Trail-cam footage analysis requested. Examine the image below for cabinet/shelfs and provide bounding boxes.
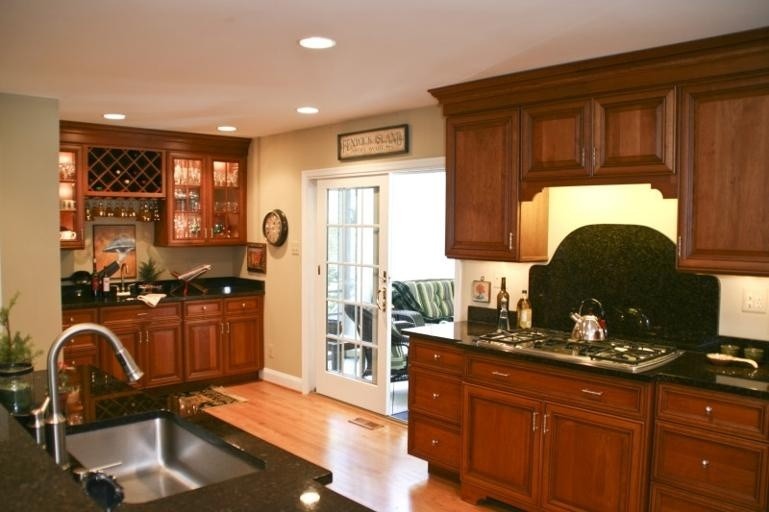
[57,120,253,248]
[99,302,184,394]
[591,83,677,184]
[444,108,548,263]
[407,333,463,483]
[519,97,592,186]
[183,292,264,391]
[675,73,769,278]
[62,307,99,372]
[649,380,769,512]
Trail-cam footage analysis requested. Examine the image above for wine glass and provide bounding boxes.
[85,198,162,224]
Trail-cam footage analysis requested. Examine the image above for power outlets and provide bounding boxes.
[742,295,763,312]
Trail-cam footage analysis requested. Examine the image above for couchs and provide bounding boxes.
[392,278,455,333]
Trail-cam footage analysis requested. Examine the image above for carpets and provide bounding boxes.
[177,382,247,409]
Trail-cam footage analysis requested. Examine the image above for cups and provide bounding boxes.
[62,199,77,209]
[743,347,764,365]
[60,230,76,240]
[175,160,232,187]
[722,343,740,356]
[177,190,238,212]
[173,214,232,238]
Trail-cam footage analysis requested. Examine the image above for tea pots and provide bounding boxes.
[568,297,609,343]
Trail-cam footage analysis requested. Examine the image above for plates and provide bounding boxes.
[103,245,136,253]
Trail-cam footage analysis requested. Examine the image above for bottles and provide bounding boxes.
[498,297,511,330]
[102,265,112,297]
[517,289,533,329]
[497,277,510,316]
[91,259,100,297]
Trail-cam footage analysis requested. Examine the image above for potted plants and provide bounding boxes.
[0,288,44,413]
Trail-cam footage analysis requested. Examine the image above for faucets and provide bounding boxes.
[44,321,144,471]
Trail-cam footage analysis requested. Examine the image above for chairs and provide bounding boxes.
[327,320,360,370]
[461,351,654,511]
[345,305,410,383]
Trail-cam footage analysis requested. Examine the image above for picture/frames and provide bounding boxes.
[472,280,491,304]
[337,123,409,162]
[93,224,137,280]
[246,243,266,274]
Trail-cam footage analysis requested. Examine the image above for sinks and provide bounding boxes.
[64,409,266,504]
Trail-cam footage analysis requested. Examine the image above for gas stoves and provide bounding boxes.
[472,325,686,376]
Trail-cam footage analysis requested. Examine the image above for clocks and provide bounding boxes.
[262,209,288,246]
